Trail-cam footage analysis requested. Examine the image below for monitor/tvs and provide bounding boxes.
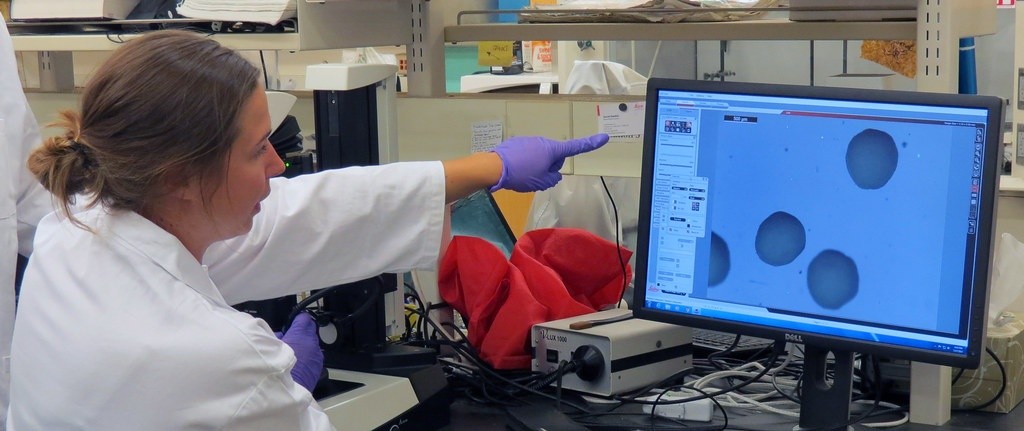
[632,76,1007,431]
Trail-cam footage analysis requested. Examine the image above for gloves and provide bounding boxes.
[487,134,609,193]
[273,313,324,394]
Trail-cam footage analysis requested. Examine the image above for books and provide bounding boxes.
[176,0,297,25]
[11,1,142,20]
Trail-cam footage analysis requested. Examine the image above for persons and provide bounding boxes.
[0,9,609,431]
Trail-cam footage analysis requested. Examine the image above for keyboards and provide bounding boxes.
[690,327,777,361]
